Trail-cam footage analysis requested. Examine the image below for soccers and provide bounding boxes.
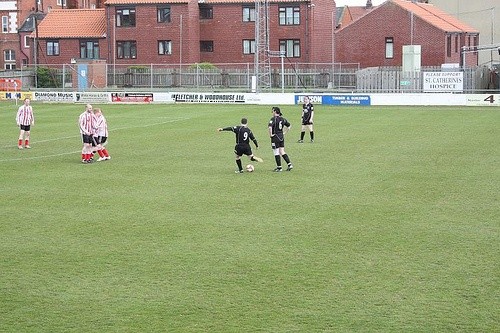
[246,163,255,172]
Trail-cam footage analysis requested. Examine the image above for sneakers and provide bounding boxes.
[252,156,263,163]
[235,169,244,173]
[285,164,293,171]
[273,167,282,172]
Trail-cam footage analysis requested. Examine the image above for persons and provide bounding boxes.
[268,107,293,172]
[16,98,35,150]
[77,104,111,164]
[296,97,315,143]
[217,117,263,174]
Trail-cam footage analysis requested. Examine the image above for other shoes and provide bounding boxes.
[311,139,314,143]
[97,156,105,162]
[297,140,303,143]
[87,159,91,163]
[90,158,93,161]
[82,159,86,162]
[18,146,23,149]
[25,146,31,148]
[105,156,110,160]
